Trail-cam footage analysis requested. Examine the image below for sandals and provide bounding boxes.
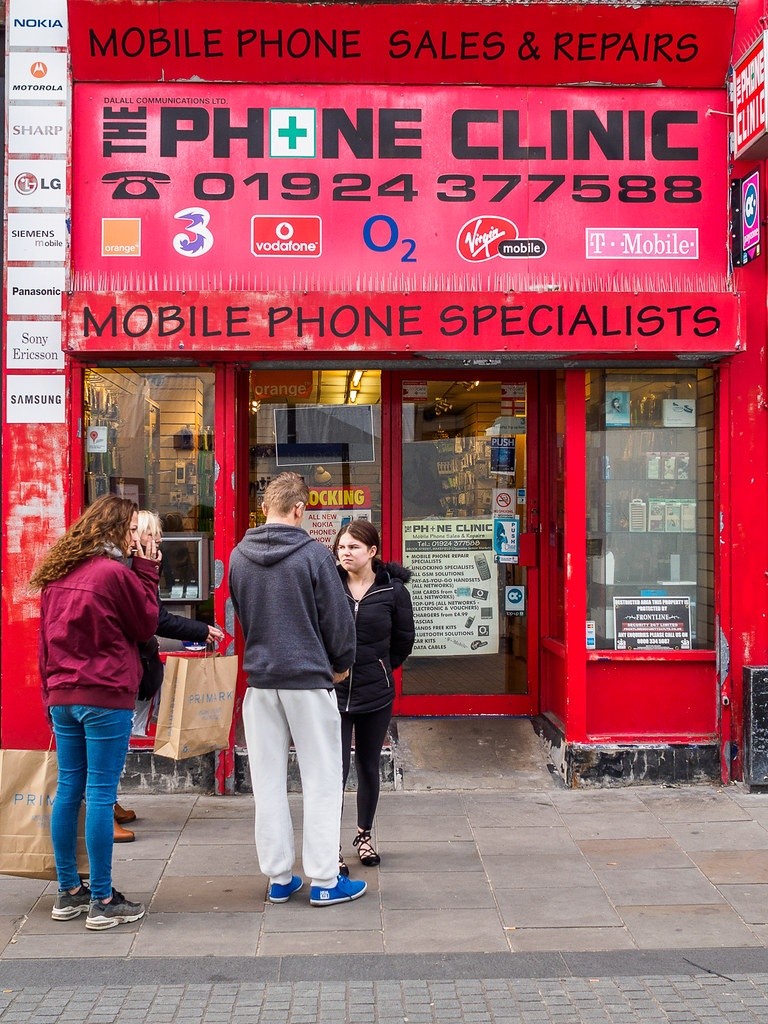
[339,853,349,877]
[353,829,380,866]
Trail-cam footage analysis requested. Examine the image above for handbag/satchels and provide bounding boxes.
[153,640,241,760]
[0,730,89,881]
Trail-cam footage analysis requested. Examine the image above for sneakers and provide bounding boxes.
[269,876,303,903]
[310,873,367,906]
[86,887,145,930]
[52,882,92,921]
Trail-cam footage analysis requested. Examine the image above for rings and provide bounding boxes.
[151,556,156,558]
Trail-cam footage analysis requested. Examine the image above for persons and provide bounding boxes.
[112,509,225,843]
[28,493,162,930]
[228,471,368,906]
[333,518,416,877]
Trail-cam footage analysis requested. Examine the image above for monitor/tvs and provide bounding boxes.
[156,536,209,601]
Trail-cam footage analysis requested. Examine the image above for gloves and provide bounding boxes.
[137,636,163,701]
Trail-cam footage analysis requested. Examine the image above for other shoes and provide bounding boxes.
[113,818,135,843]
[114,802,136,824]
